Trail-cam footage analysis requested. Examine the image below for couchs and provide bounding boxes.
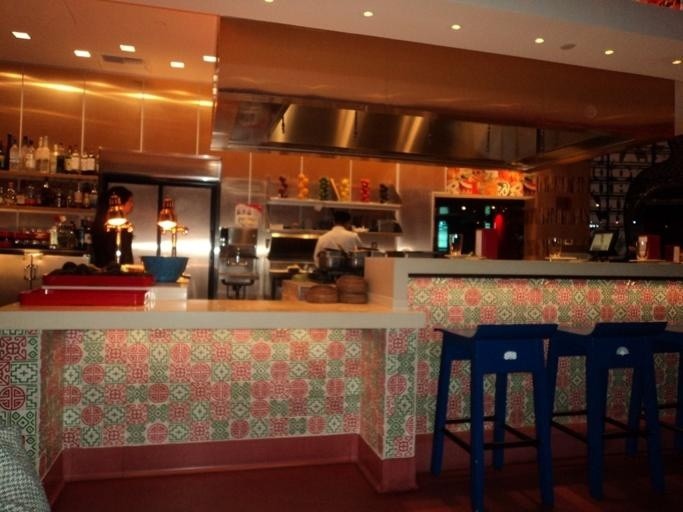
[150,276,187,300]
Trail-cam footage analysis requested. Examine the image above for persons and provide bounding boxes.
[313,210,362,268]
[92,186,136,266]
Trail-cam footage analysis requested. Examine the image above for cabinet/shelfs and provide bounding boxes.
[263,175,404,300]
[0,61,218,169]
[534,175,590,260]
[0,170,99,305]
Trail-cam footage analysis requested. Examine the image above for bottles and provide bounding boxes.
[0,130,100,176]
[0,213,96,252]
[0,179,99,209]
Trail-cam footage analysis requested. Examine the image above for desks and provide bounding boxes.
[282,280,335,300]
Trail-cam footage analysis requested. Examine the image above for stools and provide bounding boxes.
[626,330,683,457]
[545,322,668,501]
[430,324,559,512]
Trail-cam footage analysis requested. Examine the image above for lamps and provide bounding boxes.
[103,192,134,263]
[156,197,191,280]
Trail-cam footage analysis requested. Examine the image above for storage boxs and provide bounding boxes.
[18,288,148,306]
[41,273,155,286]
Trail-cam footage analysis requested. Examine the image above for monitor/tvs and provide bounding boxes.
[588,229,619,257]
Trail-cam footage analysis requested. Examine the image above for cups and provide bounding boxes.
[448,233,464,256]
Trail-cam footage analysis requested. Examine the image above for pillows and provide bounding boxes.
[0,423,51,512]
